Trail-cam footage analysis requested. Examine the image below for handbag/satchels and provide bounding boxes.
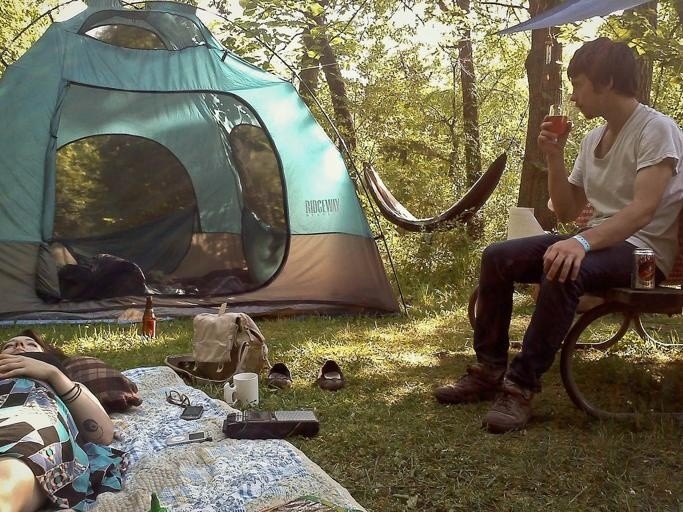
[193,303,268,380]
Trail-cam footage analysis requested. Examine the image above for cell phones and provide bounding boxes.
[180,406,203,420]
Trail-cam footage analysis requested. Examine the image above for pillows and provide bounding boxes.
[62,357,140,414]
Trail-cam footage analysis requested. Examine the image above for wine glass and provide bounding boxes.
[548,104,570,145]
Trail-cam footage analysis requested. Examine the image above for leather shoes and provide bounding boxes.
[318,359,343,390]
[267,363,293,389]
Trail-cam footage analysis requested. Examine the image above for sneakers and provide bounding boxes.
[483,380,535,431]
[435,362,506,404]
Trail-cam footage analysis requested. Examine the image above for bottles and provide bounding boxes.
[142,295,157,339]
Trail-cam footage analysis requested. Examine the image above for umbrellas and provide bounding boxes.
[489,0,655,35]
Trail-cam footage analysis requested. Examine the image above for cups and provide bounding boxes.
[223,372,259,409]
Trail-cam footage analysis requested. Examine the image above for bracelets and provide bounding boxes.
[571,235,591,254]
[60,383,82,404]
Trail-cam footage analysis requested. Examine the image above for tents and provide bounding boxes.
[0,0,401,323]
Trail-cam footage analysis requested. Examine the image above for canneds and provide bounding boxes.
[632,248,656,290]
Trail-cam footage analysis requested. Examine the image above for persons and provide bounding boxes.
[0,328,114,512]
[433,38,683,434]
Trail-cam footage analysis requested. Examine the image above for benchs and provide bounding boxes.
[468,279,680,423]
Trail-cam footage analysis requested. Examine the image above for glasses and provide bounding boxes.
[165,391,189,408]
